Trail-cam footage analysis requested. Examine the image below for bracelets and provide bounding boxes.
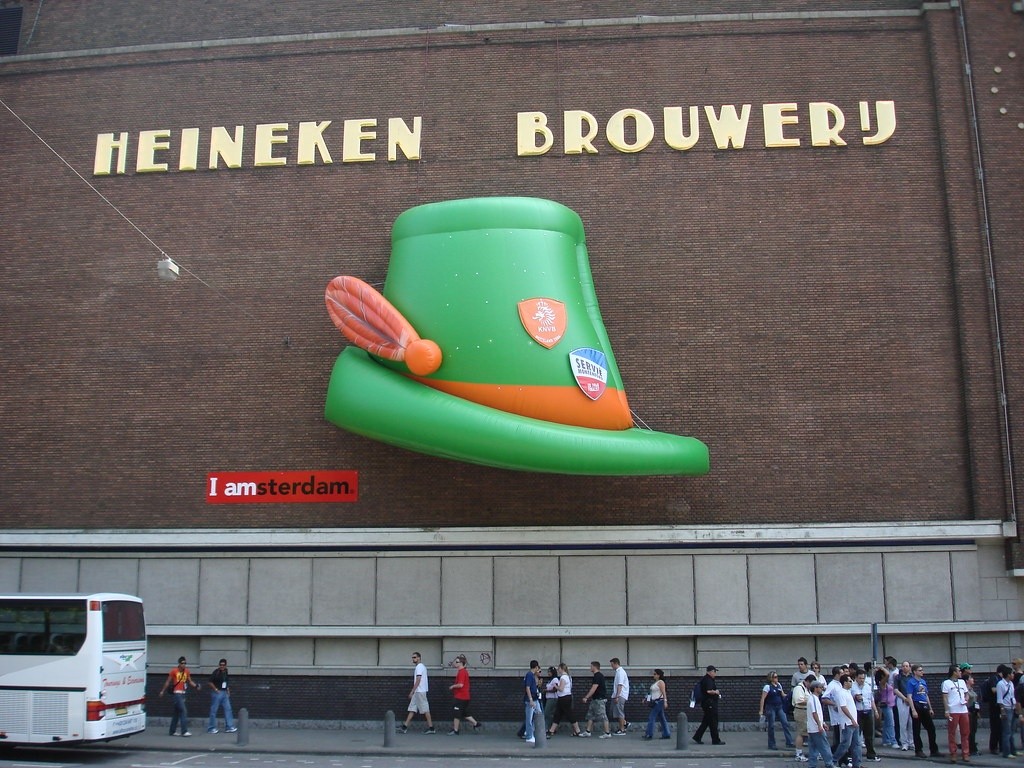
[215,688,218,692]
[585,696,589,699]
[664,699,667,701]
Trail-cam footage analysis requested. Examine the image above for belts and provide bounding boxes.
[795,707,807,710]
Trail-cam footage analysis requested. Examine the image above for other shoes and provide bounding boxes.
[525,722,726,745]
[182,732,192,737]
[517,731,526,739]
[172,732,179,735]
[763,736,1024,768]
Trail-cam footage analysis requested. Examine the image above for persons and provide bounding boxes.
[986,657,1024,758]
[446,657,482,736]
[523,660,543,742]
[609,658,632,735]
[642,669,670,739]
[546,662,580,736]
[894,660,946,757]
[160,656,201,737]
[692,665,726,745]
[578,661,612,738]
[517,666,551,739]
[941,662,982,762]
[790,655,900,768]
[395,652,436,735]
[758,670,796,749]
[207,659,238,734]
[544,667,559,734]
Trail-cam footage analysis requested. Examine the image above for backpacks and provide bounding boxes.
[782,685,804,714]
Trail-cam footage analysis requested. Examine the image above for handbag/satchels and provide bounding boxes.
[520,678,526,692]
[168,693,177,705]
[759,714,766,732]
[648,703,655,708]
[689,679,702,708]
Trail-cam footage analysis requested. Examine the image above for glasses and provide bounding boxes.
[552,668,555,670]
[1011,662,1017,666]
[412,657,416,659]
[219,663,226,666]
[918,670,922,671]
[456,661,460,663]
[849,680,852,682]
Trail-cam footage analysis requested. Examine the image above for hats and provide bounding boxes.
[706,665,719,672]
[960,662,973,670]
[179,657,185,662]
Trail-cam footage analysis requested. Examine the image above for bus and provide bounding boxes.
[0,592,147,754]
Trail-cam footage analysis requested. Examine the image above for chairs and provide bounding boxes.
[10,632,74,654]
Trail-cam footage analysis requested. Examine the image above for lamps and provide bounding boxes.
[156,253,180,282]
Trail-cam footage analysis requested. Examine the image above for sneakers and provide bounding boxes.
[424,729,435,734]
[208,729,219,734]
[474,722,482,735]
[446,729,460,736]
[225,726,237,733]
[395,726,408,734]
